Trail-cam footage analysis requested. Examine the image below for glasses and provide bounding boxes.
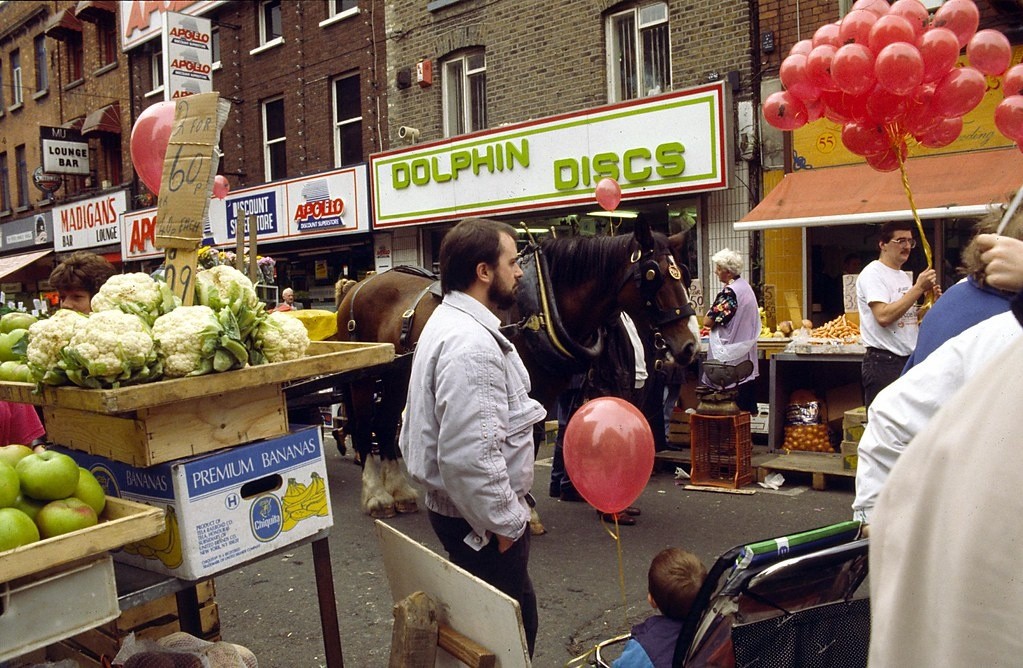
[889,238,917,249]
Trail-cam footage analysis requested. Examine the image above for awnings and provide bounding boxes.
[45,0,115,41]
[59,105,122,136]
[0,248,52,279]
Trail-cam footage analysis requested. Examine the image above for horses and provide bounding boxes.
[335,207,700,534]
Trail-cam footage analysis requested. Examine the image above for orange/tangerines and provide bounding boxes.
[781,398,834,453]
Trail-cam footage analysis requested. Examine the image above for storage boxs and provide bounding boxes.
[45,577,224,668]
[840,440,859,471]
[690,410,751,490]
[53,422,336,582]
[842,406,867,442]
[43,384,290,468]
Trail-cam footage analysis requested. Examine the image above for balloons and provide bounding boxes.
[763,0,1023,173]
[563,397,657,520]
[213,176,229,199]
[595,178,622,211]
[130,101,179,197]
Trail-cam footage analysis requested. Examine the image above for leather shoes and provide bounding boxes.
[598,504,640,525]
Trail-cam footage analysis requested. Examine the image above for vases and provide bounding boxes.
[137,197,151,207]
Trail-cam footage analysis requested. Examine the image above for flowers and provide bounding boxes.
[134,187,153,205]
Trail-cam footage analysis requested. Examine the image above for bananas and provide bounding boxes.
[757,326,772,339]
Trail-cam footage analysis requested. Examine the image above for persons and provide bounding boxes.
[49,251,118,316]
[277,288,303,311]
[0,400,46,450]
[701,248,762,415]
[851,197,1023,668]
[627,53,653,99]
[608,550,707,668]
[663,263,701,451]
[399,215,547,664]
[550,377,641,525]
[331,279,362,465]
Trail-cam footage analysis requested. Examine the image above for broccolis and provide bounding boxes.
[11,264,309,387]
